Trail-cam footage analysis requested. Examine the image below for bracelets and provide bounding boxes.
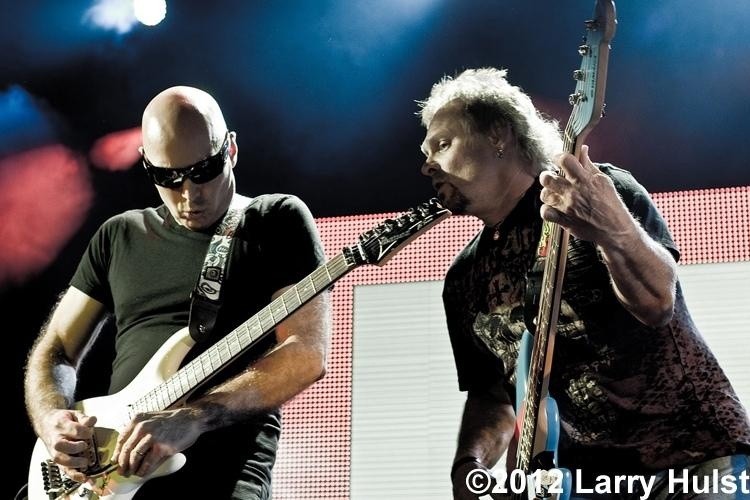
[450,455,482,482]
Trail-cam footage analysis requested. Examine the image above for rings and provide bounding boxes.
[133,448,144,459]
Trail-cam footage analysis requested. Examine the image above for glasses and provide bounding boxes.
[141,128,231,190]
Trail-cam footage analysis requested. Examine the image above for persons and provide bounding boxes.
[417,66,749,500]
[20,85,327,500]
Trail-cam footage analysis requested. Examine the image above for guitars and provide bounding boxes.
[505,2,618,498]
[27,197,452,499]
